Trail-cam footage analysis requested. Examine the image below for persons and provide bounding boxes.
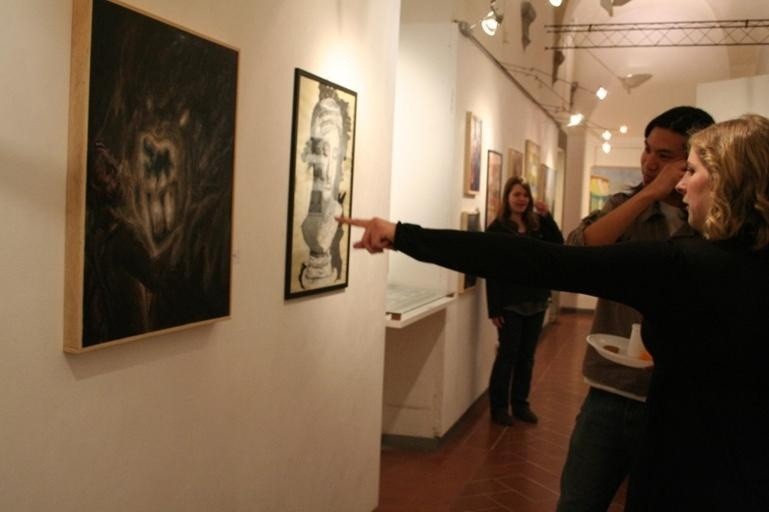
[333,112,769,512]
[482,175,566,426]
[557,104,719,511]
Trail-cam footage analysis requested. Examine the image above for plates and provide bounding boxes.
[585,332,655,368]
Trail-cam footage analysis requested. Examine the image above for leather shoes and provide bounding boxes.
[513,405,537,423]
[493,413,512,426]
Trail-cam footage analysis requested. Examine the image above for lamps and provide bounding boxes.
[585,48,652,93]
[499,61,628,153]
[469,10,502,37]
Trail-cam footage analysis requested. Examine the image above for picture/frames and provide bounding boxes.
[286,68,358,299]
[63,0,240,356]
[458,110,553,294]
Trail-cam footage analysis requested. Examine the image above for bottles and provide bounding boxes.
[628,324,646,358]
